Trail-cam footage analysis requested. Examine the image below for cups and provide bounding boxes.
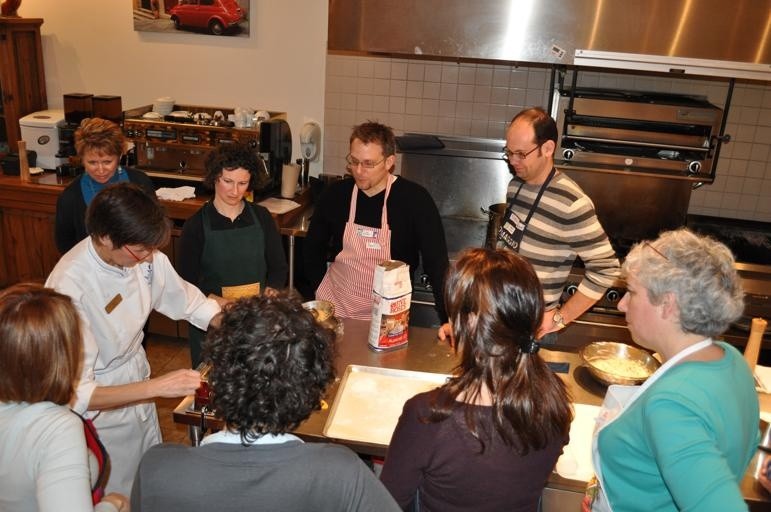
[280,164,300,199]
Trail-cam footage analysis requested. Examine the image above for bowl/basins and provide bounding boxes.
[303,300,336,324]
[579,340,661,388]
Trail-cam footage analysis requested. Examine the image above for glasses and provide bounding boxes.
[642,242,668,260]
[123,245,156,262]
[346,153,390,168]
[502,144,542,159]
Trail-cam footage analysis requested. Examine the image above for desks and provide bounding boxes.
[173,314,771,512]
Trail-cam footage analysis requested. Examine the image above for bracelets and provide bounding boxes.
[99,494,124,510]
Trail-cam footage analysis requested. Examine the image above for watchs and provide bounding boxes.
[554,309,568,329]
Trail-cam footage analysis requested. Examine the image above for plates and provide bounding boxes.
[143,95,176,121]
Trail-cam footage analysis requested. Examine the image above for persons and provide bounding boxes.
[129,296,405,512]
[55,119,159,254]
[380,249,575,512]
[590,227,762,512]
[1,283,126,510]
[296,123,459,350]
[178,146,288,370]
[496,107,623,352]
[44,184,225,510]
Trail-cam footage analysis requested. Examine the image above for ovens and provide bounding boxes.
[560,95,723,187]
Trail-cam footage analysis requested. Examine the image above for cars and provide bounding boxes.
[168,0,246,35]
